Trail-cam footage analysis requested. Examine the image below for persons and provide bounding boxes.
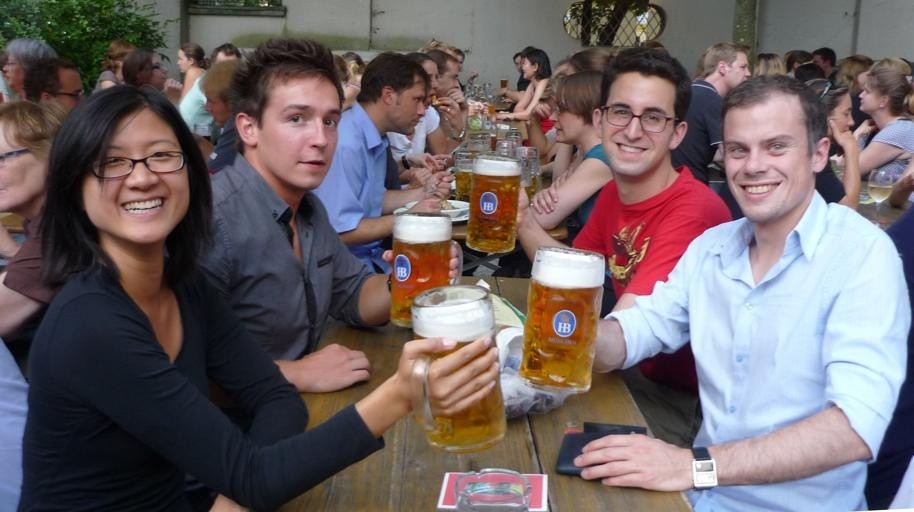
[494,41,913,510]
[0,36,500,512]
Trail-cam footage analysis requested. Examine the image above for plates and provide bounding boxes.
[406,199,470,217]
[394,205,469,222]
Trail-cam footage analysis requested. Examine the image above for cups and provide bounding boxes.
[447,129,543,200]
[465,153,522,254]
[499,78,508,88]
[410,285,507,455]
[389,214,453,328]
[518,245,606,394]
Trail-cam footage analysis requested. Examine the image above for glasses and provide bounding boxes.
[151,62,164,70]
[90,151,186,180]
[51,88,84,101]
[0,148,30,162]
[819,81,834,101]
[554,101,576,115]
[601,104,678,133]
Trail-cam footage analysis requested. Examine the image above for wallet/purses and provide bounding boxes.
[556,422,646,476]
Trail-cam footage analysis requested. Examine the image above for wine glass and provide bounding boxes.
[866,169,894,218]
